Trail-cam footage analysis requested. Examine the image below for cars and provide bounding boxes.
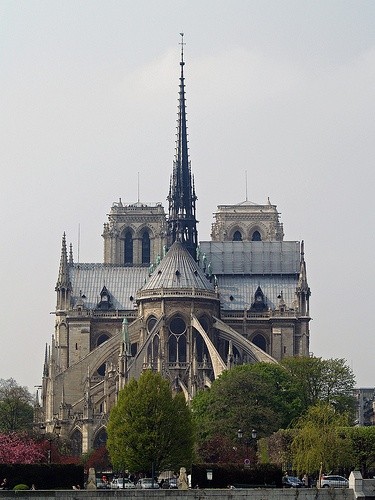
[83,478,110,489]
[109,478,136,489]
[136,478,160,489]
[282,476,305,488]
[162,478,178,489]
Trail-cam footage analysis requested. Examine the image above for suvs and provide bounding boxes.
[320,474,349,488]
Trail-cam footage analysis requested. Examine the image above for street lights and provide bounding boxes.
[38,423,61,463]
[237,429,257,458]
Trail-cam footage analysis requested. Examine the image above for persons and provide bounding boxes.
[160,479,164,488]
[302,474,308,488]
